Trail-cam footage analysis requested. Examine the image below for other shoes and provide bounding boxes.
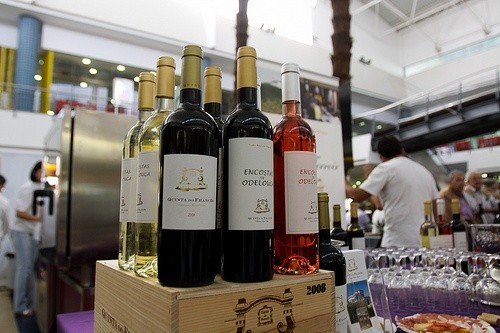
[17,309,32,318]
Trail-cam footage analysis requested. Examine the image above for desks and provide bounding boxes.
[55,301,500,333]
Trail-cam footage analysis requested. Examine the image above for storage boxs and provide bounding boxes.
[94,259,336,333]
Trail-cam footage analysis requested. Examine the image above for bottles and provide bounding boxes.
[220,45,274,284]
[202,67,225,276]
[271,62,320,276]
[419,199,439,266]
[449,198,473,276]
[355,291,373,330]
[118,72,156,272]
[435,199,456,270]
[329,205,350,251]
[156,45,220,288]
[132,55,176,281]
[346,201,366,250]
[318,191,348,333]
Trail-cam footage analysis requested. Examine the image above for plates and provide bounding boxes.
[396,312,496,333]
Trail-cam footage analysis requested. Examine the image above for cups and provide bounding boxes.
[470,224,500,254]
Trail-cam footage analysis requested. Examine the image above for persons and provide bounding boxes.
[346,134,439,266]
[439,170,483,225]
[463,170,498,224]
[0,175,12,241]
[346,202,370,229]
[9,161,43,316]
[369,195,384,233]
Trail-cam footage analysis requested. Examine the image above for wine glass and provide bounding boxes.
[361,246,500,310]
